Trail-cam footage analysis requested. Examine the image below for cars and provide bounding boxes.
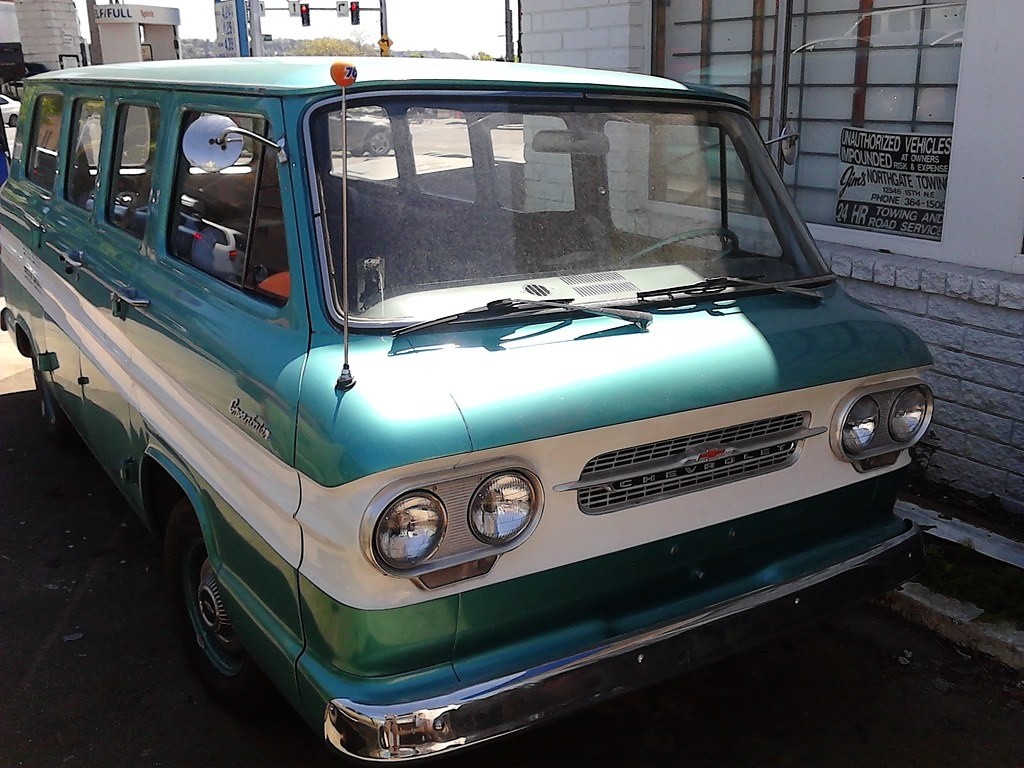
[326,111,396,157]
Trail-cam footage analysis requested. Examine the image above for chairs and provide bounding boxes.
[507,211,625,277]
[209,198,381,293]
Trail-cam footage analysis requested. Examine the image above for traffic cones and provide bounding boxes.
[456,110,460,120]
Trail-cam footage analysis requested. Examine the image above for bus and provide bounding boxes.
[2,51,943,767]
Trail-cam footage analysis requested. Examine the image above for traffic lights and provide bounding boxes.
[351,2,361,25]
[300,4,311,27]
